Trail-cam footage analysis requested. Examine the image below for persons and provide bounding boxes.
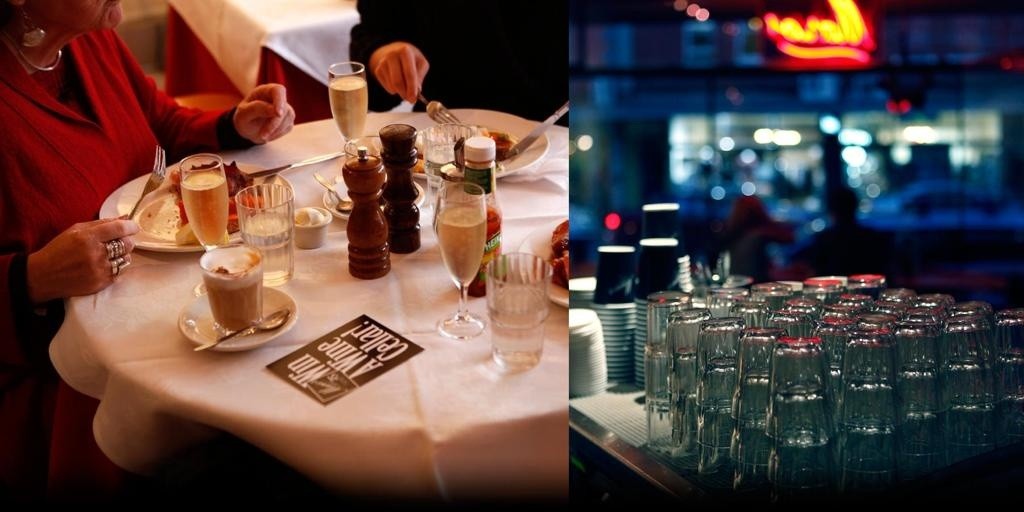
[704,194,810,279]
[791,185,886,276]
[348,1,569,128]
[0,1,302,510]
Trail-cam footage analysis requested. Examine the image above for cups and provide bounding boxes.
[295,208,333,249]
[423,124,470,192]
[644,267,1022,452]
[198,245,265,336]
[644,405,1021,493]
[569,197,693,398]
[484,252,554,368]
[234,183,296,290]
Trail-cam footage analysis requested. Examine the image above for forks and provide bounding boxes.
[413,90,460,127]
[128,145,169,221]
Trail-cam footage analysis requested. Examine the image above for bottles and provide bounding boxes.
[342,125,423,278]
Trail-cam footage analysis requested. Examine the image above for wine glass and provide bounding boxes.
[433,182,486,338]
[179,153,230,297]
[328,62,368,146]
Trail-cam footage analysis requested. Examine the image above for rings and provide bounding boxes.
[106,239,127,259]
[109,256,130,284]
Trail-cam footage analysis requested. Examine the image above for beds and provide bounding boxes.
[60,107,568,501]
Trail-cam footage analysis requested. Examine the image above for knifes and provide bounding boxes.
[245,151,345,182]
[505,97,571,161]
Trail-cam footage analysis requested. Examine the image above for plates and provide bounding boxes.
[519,215,569,307]
[473,123,549,182]
[178,285,298,352]
[98,164,295,253]
[322,179,425,220]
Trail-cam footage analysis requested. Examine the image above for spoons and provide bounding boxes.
[191,303,293,352]
[313,170,352,213]
